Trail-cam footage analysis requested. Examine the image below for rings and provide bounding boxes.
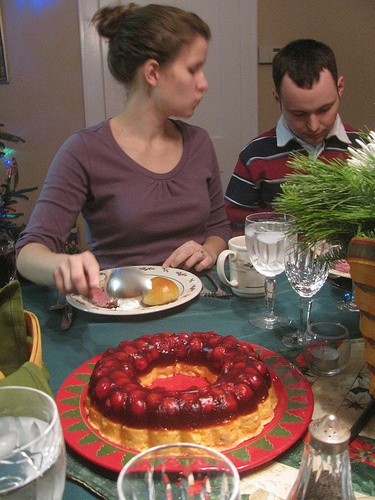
[197,249,206,259]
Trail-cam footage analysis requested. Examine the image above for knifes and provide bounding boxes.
[61,299,73,330]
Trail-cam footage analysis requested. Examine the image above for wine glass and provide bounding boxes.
[282,232,332,347]
[337,279,360,312]
[245,212,297,329]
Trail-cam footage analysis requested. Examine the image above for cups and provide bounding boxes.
[0,386,66,500]
[217,235,266,298]
[0,230,17,289]
[303,322,351,376]
[117,443,240,500]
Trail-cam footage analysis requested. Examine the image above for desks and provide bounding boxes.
[20,264,375,500]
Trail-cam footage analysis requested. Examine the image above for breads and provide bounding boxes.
[141,277,179,305]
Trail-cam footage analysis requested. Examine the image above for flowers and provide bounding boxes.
[269,123,375,263]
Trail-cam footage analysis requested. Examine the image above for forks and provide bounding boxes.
[194,268,214,297]
[203,270,232,297]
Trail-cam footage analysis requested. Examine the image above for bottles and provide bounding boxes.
[288,413,356,500]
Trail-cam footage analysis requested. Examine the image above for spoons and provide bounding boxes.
[50,289,64,311]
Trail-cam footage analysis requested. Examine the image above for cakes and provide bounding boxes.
[83,331,278,456]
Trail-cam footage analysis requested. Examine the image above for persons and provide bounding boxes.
[15,3,232,296]
[223,39,374,239]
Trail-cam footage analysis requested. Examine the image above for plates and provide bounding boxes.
[66,265,202,315]
[329,268,351,279]
[56,341,314,473]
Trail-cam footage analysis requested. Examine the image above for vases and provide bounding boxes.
[347,236,375,400]
